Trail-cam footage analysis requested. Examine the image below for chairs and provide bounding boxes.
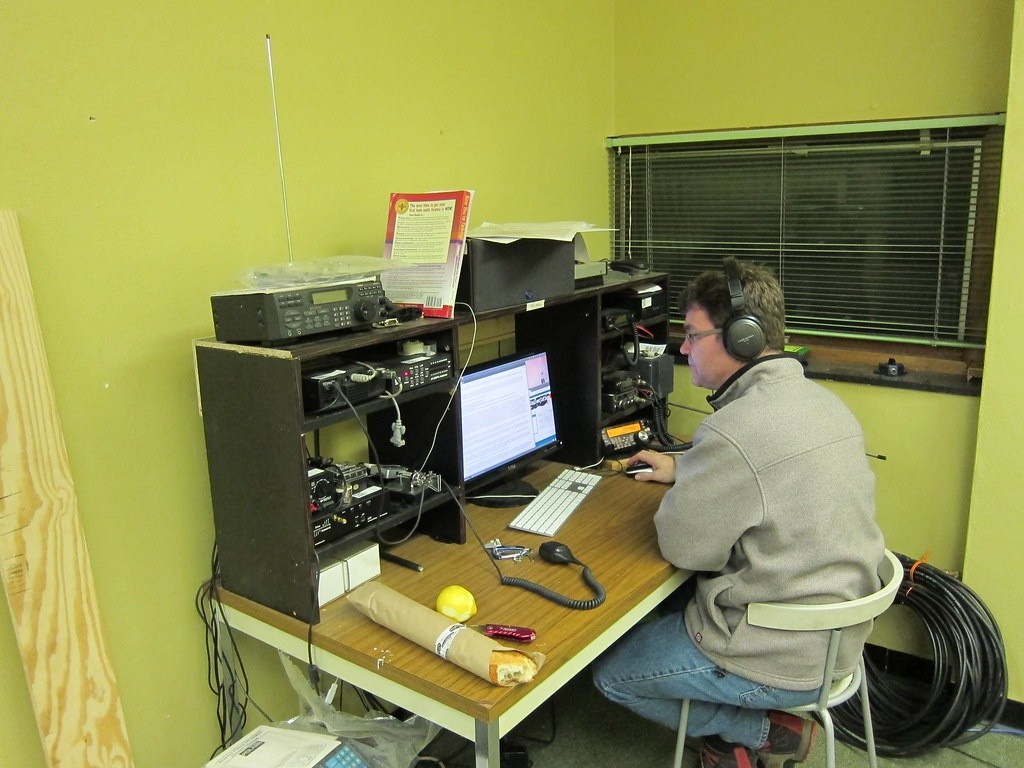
[673,545,903,768]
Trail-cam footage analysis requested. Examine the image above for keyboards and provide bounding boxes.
[509,469,602,537]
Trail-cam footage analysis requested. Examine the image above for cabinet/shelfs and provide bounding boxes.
[193,271,672,625]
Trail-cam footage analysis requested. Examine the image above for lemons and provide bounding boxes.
[436,585,477,622]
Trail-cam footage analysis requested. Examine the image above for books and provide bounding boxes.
[380,191,470,317]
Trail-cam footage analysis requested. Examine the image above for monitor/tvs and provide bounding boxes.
[461,344,565,507]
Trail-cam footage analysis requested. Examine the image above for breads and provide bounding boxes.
[489,650,538,686]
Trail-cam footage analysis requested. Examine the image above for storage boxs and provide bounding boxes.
[326,539,381,593]
[315,556,345,609]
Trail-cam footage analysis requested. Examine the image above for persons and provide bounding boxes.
[594,257,885,768]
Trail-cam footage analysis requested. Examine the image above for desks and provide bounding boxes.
[202,433,696,768]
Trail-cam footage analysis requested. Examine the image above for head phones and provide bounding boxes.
[721,255,766,362]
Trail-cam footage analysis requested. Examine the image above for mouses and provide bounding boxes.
[626,461,653,477]
